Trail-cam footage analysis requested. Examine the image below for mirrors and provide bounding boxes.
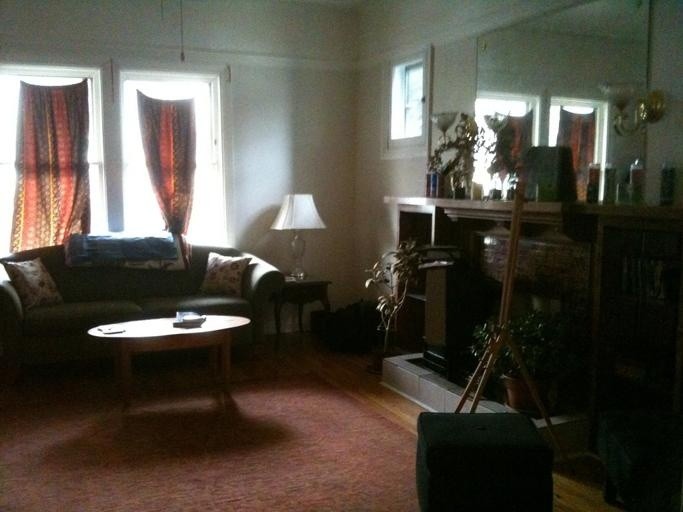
[468,0,651,201]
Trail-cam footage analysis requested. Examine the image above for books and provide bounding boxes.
[425,173,453,198]
[173,311,206,328]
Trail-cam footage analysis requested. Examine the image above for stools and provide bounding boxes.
[414,412,555,512]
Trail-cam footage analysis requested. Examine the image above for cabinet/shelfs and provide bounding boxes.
[392,206,465,360]
[587,214,682,420]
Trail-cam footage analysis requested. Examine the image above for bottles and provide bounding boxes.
[426,173,441,198]
[587,157,676,207]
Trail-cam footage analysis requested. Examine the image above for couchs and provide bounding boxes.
[0,245,284,385]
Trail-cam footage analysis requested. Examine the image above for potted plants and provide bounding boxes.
[364,238,419,374]
[469,310,564,416]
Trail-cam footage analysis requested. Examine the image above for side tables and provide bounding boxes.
[275,273,332,353]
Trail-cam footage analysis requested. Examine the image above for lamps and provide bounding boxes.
[597,80,663,136]
[271,194,328,277]
[430,110,469,148]
[475,112,509,154]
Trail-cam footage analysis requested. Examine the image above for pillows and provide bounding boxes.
[4,257,65,312]
[199,252,252,298]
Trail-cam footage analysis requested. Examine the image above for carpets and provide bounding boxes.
[0,352,419,512]
